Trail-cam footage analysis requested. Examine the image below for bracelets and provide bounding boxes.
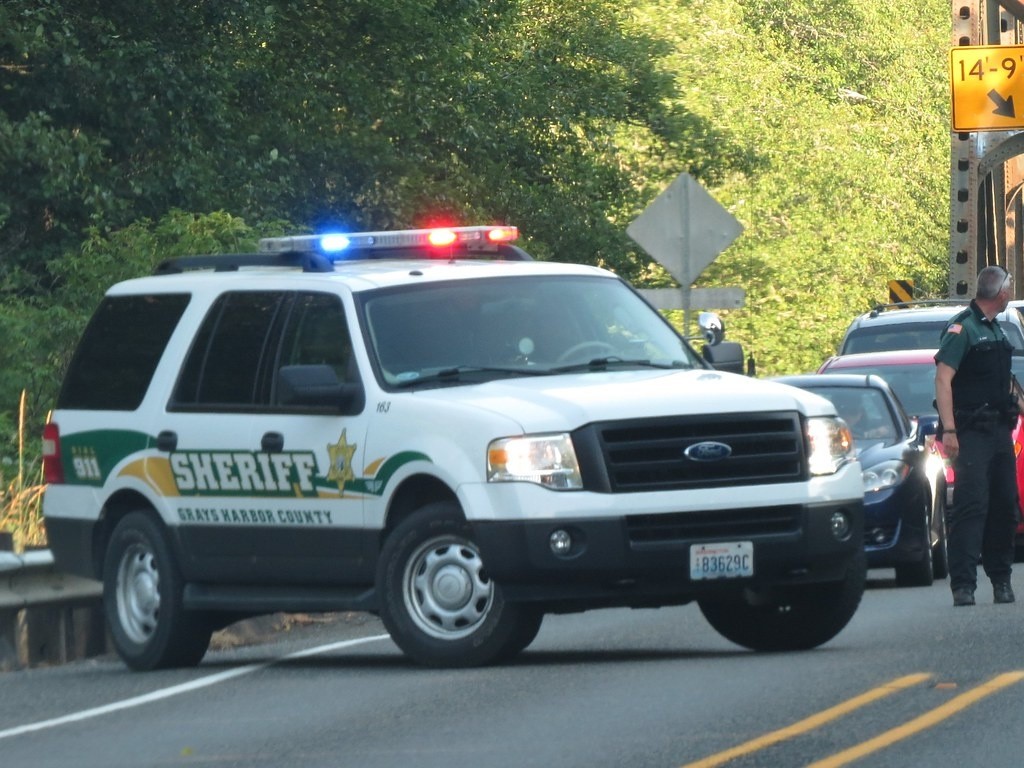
[942,429,957,434]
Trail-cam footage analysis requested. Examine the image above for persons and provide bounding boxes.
[933,266,1024,607]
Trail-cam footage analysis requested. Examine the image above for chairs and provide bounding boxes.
[880,379,913,416]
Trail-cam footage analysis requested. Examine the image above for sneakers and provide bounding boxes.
[992,579,1015,604]
[951,587,976,606]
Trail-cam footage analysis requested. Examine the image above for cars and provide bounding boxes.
[765,372,948,588]
[815,349,1023,563]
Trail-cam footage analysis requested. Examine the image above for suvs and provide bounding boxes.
[840,298,1023,391]
[42,224,868,670]
